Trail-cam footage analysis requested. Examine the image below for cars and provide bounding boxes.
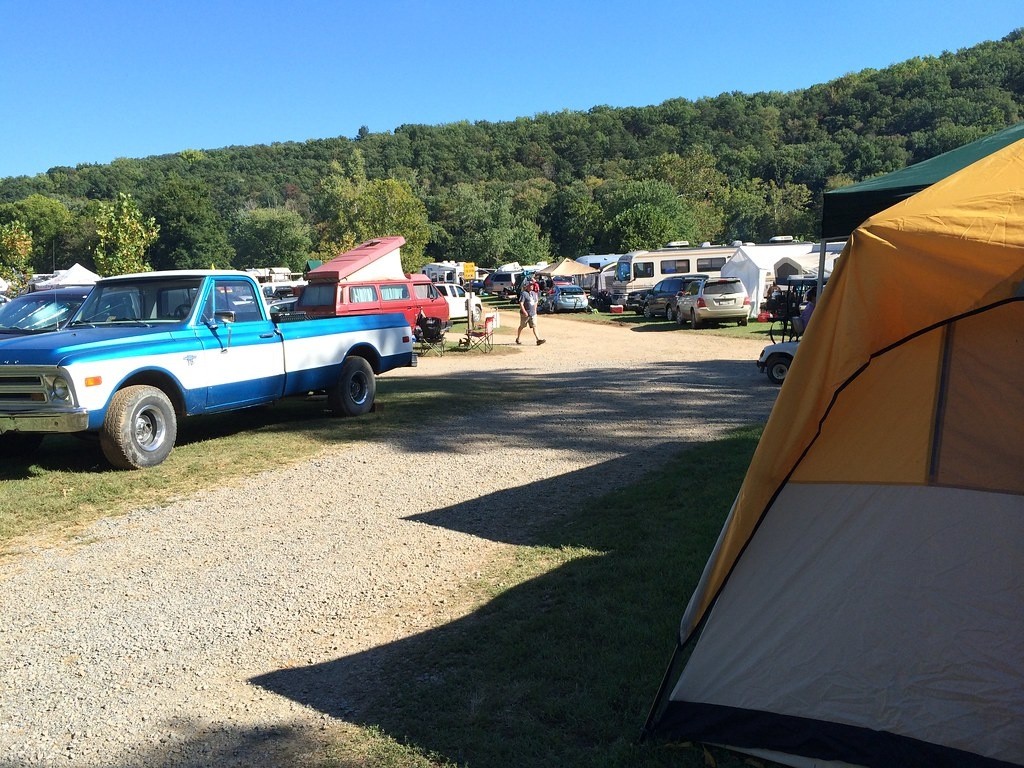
[756,340,800,384]
[462,270,591,313]
[217,284,299,312]
[625,287,652,315]
[435,283,484,322]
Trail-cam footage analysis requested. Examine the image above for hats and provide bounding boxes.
[526,281,534,286]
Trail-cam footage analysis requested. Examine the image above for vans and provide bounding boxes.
[293,232,449,342]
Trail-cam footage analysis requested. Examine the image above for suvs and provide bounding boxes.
[675,277,752,330]
[640,273,710,323]
[0,286,141,329]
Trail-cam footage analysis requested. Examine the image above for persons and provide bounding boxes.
[800,287,817,332]
[514,281,546,346]
[529,278,539,316]
[537,275,556,292]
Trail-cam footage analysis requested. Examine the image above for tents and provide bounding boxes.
[817,122,1024,299]
[34,261,103,292]
[640,134,1024,768]
[535,256,603,298]
[719,243,841,320]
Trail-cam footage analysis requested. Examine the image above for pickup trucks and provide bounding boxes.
[0,268,419,471]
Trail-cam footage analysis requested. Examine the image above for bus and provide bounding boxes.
[610,235,815,306]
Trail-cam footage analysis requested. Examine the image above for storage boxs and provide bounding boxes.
[610,305,623,313]
[758,313,773,322]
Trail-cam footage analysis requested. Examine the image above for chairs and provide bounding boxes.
[466,315,494,354]
[419,317,445,357]
[98,298,128,321]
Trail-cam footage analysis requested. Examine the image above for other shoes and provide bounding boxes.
[515,338,521,344]
[537,339,546,346]
